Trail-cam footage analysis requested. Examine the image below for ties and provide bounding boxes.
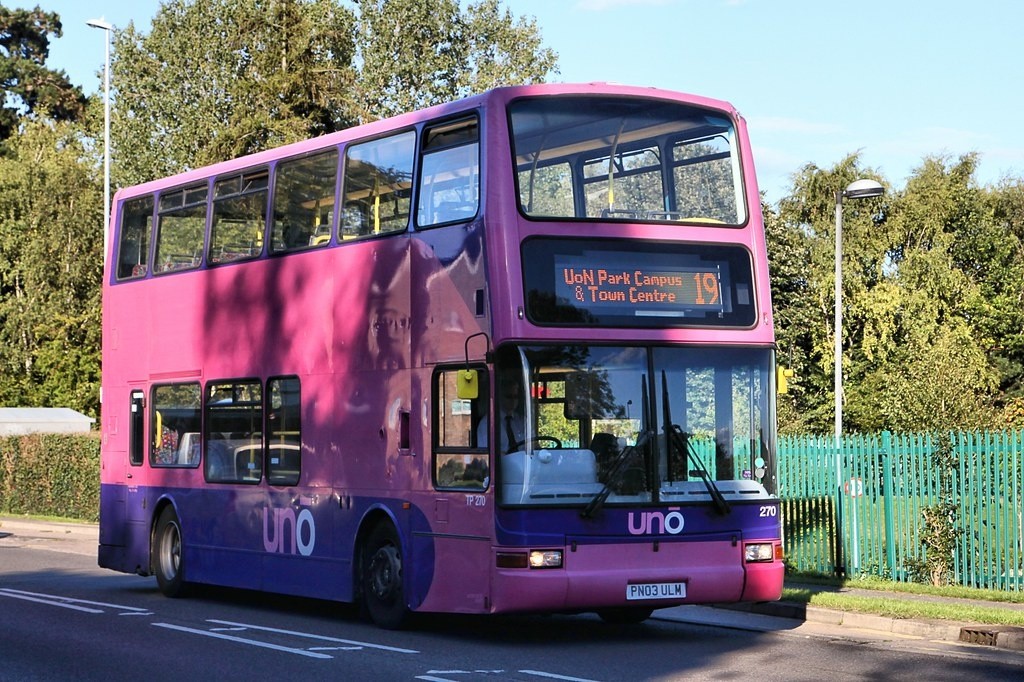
[504,414,520,452]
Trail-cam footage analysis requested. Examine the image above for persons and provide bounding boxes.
[279,177,322,249]
[477,373,541,457]
[189,416,237,480]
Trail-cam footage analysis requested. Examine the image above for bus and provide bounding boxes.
[99,81,786,628]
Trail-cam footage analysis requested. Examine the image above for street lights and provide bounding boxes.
[86,19,115,264]
[829,179,887,568]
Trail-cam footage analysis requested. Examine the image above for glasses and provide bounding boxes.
[501,393,518,399]
